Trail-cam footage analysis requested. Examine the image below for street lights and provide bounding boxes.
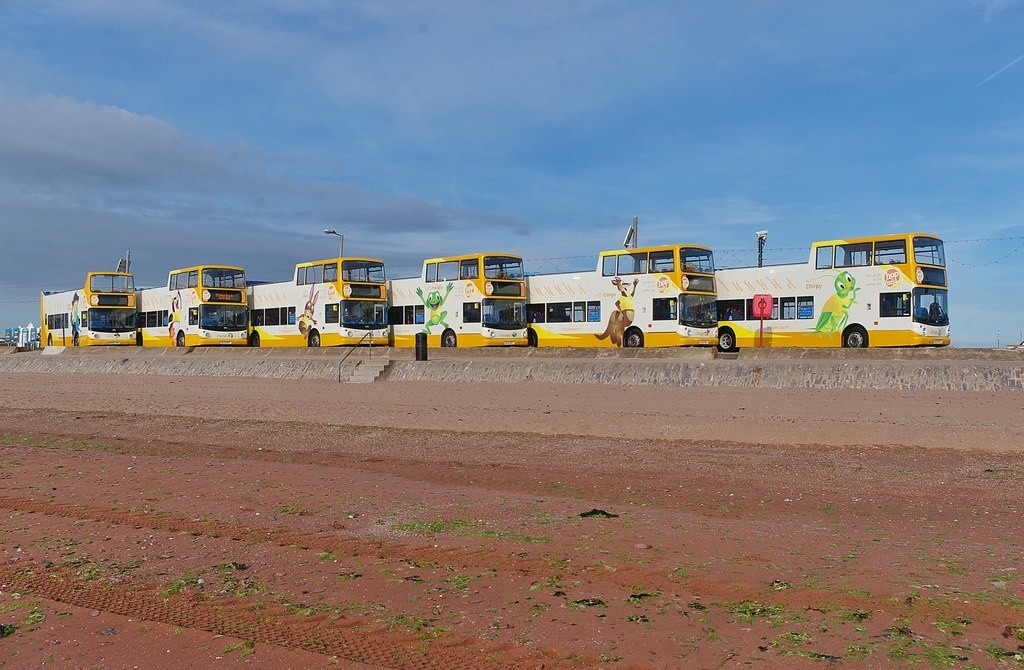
[324,228,343,258]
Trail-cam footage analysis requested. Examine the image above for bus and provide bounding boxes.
[381,251,530,350]
[710,232,952,354]
[38,271,139,347]
[521,244,719,349]
[244,257,391,348]
[132,265,249,347]
